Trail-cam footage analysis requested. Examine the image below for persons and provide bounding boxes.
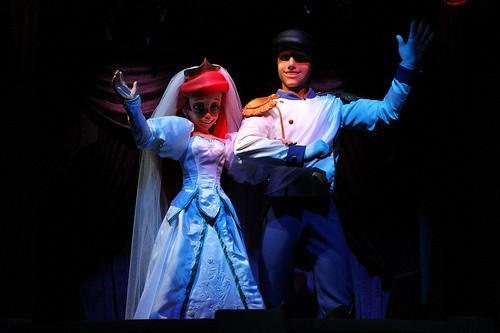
[233,19,438,321]
[110,54,297,319]
